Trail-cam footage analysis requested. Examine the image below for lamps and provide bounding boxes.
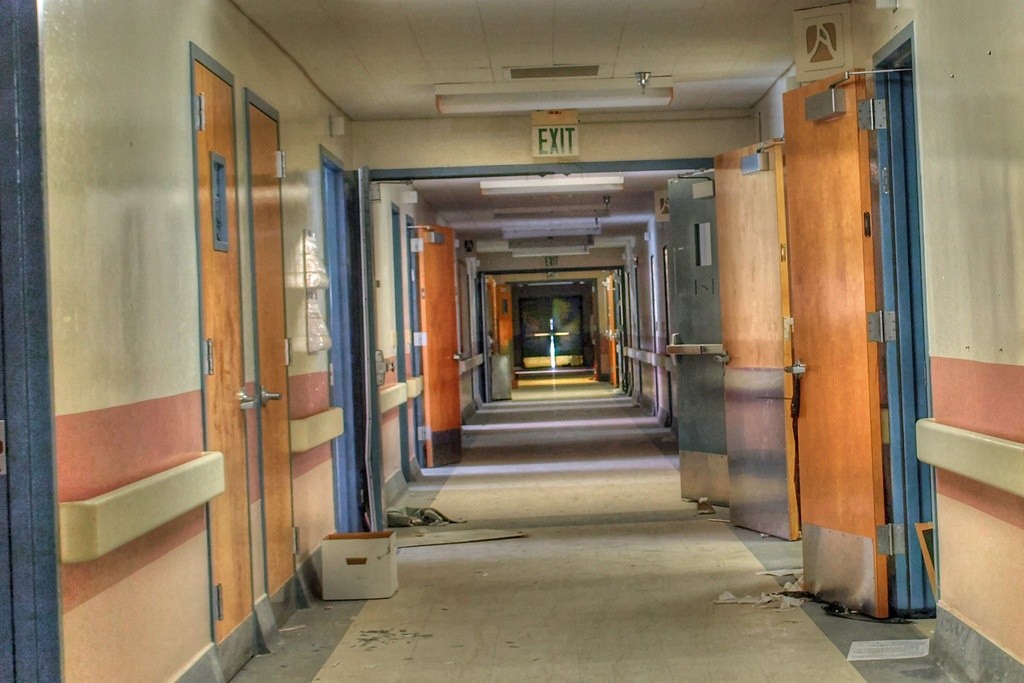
[495,196,611,256]
[435,72,675,115]
[480,172,624,195]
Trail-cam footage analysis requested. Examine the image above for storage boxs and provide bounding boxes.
[320,530,400,601]
